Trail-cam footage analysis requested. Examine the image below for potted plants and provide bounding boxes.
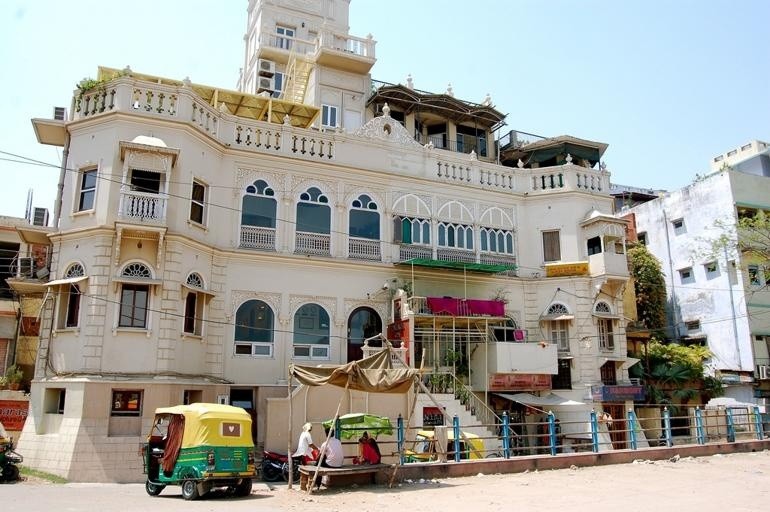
[5,363,24,390]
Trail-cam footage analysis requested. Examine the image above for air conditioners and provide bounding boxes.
[52,105,67,122]
[257,57,275,96]
[759,366,770,380]
[29,207,50,226]
[17,257,34,277]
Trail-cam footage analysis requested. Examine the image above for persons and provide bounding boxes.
[358,431,381,464]
[292,423,320,461]
[316,427,344,468]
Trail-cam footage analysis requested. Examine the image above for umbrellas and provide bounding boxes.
[321,413,393,455]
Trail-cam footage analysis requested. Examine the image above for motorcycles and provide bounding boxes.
[257,448,298,482]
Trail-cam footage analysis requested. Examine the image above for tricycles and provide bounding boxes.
[139,401,258,501]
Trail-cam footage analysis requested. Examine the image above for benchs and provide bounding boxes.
[299,460,392,492]
